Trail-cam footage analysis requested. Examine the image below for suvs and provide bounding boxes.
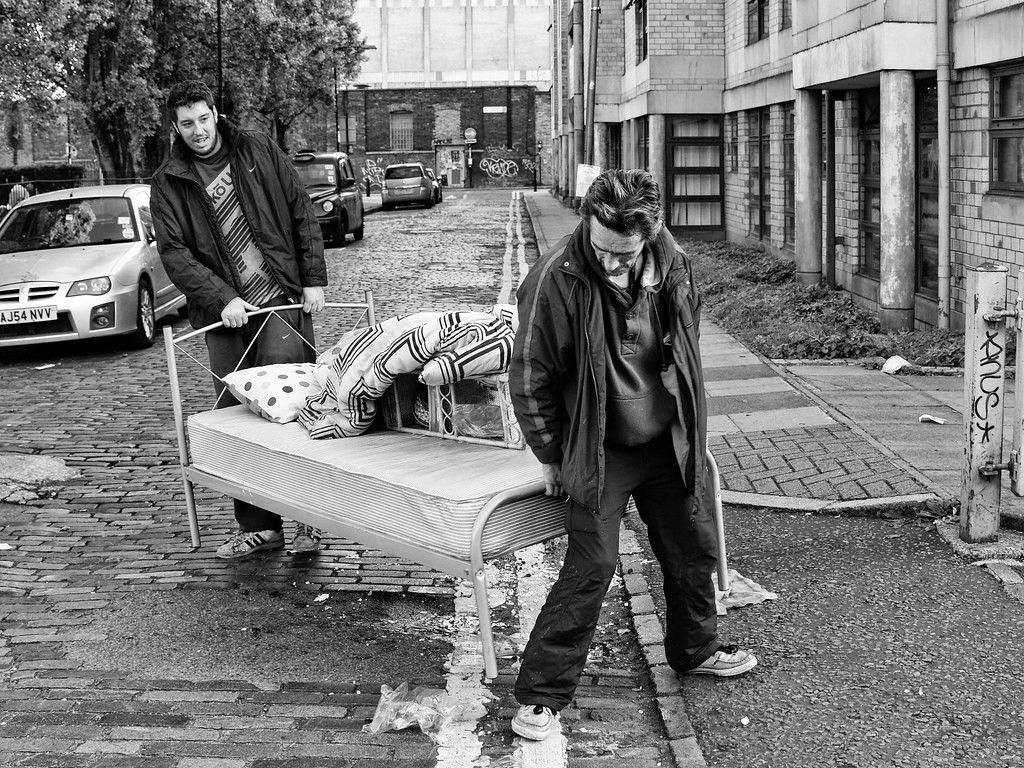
[382,162,436,208]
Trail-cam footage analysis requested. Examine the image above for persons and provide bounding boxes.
[0,173,30,221]
[149,78,328,558]
[505,167,759,742]
[135,162,145,184]
[20,174,40,197]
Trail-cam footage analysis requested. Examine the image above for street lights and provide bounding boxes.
[333,44,378,152]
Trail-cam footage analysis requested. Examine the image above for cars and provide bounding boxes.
[411,167,443,204]
[285,151,364,248]
[0,183,186,349]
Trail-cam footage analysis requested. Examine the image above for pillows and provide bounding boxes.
[219,362,330,426]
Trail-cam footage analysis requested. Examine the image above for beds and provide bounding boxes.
[162,289,736,683]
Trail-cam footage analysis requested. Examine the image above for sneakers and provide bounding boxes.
[215,526,286,557]
[511,700,558,744]
[680,638,759,679]
[290,518,324,553]
